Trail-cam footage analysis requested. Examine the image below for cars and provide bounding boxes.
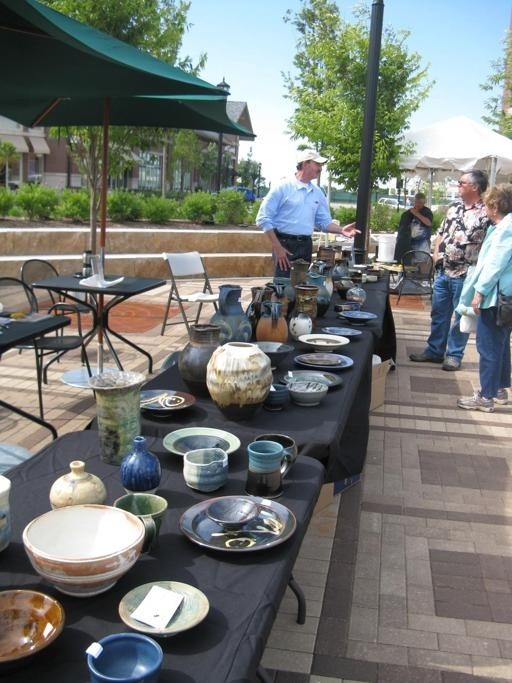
[374,194,461,211]
[218,184,256,202]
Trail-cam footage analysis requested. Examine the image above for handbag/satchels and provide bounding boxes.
[495,292,512,327]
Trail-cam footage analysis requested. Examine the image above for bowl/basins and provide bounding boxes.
[20,503,146,598]
[365,266,384,283]
[254,433,299,463]
[286,383,329,408]
[207,497,259,526]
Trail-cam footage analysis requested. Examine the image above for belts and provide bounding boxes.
[279,235,312,242]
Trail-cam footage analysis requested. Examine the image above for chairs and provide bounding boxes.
[378,249,435,305]
[160,250,219,337]
[19,259,94,362]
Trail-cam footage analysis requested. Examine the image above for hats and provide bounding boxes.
[295,148,328,164]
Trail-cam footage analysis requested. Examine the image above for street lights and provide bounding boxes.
[213,74,232,190]
[257,161,262,198]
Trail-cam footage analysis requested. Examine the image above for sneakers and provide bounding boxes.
[492,389,509,404]
[409,351,444,363]
[457,390,495,412]
[443,355,462,370]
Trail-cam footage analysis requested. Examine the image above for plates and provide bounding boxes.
[119,578,213,639]
[178,496,298,552]
[284,310,379,389]
[138,390,196,419]
[0,587,65,669]
[162,426,240,463]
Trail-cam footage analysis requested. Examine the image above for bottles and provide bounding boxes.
[264,242,367,334]
[176,285,295,420]
[121,435,162,495]
[85,368,145,466]
[48,461,106,509]
[0,470,16,554]
[81,249,93,276]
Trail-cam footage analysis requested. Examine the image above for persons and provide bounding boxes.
[406,168,498,372]
[452,183,512,412]
[394,191,436,268]
[255,147,363,281]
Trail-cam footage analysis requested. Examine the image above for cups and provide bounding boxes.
[84,631,166,683]
[114,494,168,561]
[244,439,294,498]
[182,447,230,493]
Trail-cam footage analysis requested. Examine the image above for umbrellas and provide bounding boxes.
[0,0,260,375]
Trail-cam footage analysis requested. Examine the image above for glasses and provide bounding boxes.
[456,179,472,186]
